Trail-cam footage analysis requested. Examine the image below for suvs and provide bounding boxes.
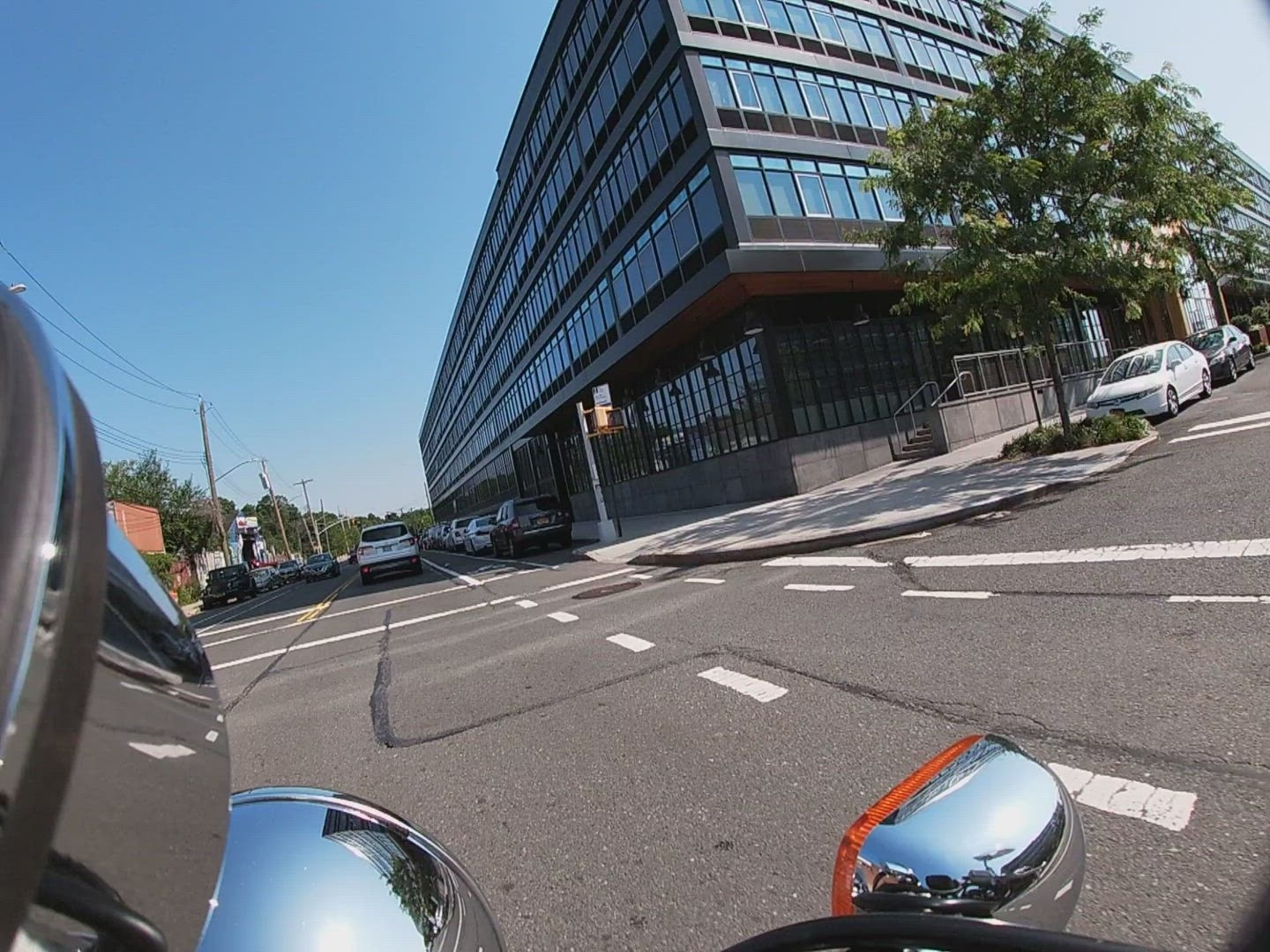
[355,522,423,586]
[198,562,261,608]
[487,494,574,559]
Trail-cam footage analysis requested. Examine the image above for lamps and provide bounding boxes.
[655,314,717,385]
[849,277,870,326]
[740,284,764,336]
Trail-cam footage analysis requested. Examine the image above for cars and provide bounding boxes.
[277,560,304,586]
[303,554,341,584]
[249,566,283,591]
[348,549,358,564]
[1181,324,1256,383]
[418,513,498,558]
[1085,340,1213,421]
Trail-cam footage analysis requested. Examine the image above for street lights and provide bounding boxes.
[275,494,301,559]
[210,457,266,567]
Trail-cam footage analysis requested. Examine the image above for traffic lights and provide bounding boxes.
[590,406,608,432]
[606,407,625,430]
